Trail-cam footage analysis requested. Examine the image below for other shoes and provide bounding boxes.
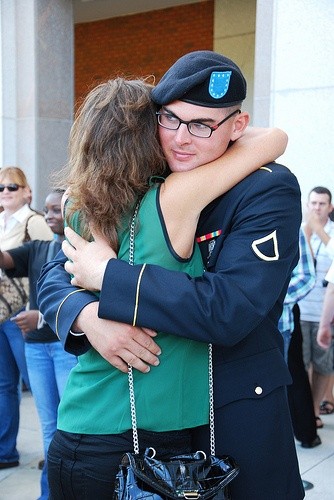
[0,461,19,469]
[302,435,321,449]
[39,460,44,470]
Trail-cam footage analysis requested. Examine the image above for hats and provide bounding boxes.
[151,51,246,106]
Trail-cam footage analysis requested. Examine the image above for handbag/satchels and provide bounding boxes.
[115,451,239,500]
[0,272,29,324]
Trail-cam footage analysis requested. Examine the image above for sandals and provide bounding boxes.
[315,416,323,429]
[320,401,334,414]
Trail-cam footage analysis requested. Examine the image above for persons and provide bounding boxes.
[0,190,80,500]
[47,78,288,499]
[0,167,54,469]
[36,48,305,500]
[278,188,334,448]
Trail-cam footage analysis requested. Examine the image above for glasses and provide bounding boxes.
[0,185,25,192]
[156,108,241,139]
